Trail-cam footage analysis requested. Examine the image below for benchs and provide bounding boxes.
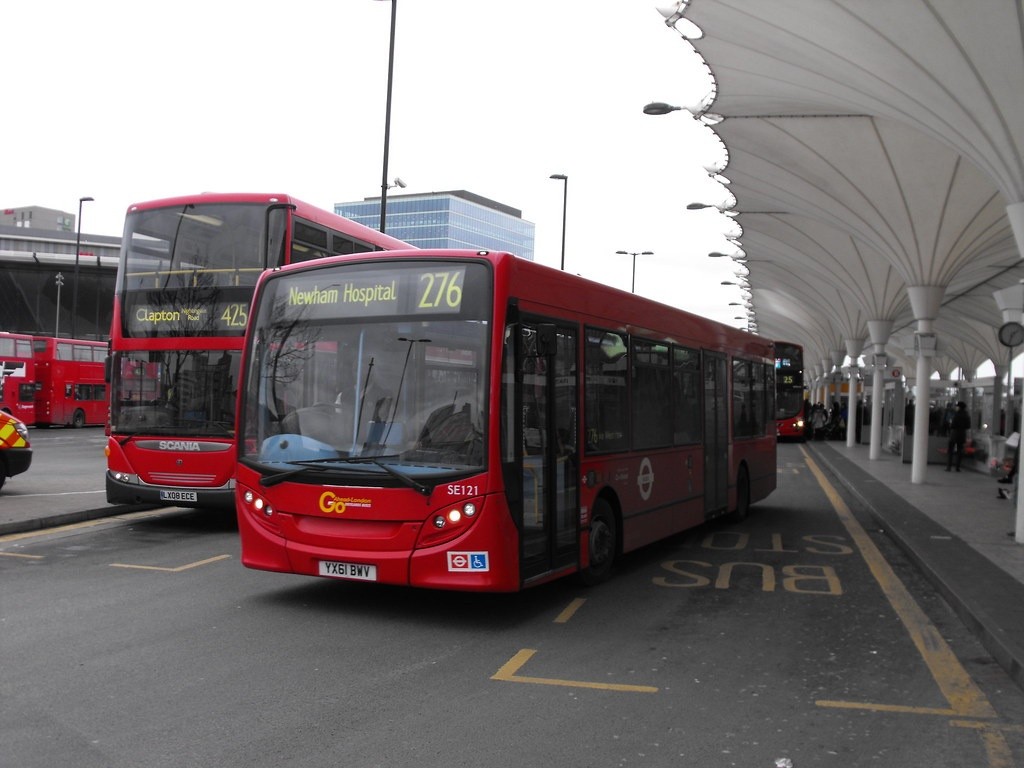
[936,436,988,463]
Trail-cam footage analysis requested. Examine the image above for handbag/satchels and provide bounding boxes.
[839,419,845,428]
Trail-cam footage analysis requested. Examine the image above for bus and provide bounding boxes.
[31,336,161,429]
[230,248,779,594]
[97,191,479,513]
[772,340,809,439]
[0,329,36,426]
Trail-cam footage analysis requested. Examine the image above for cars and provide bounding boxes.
[0,408,34,489]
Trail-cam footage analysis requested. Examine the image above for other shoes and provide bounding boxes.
[956,469,960,472]
[944,466,951,471]
[998,476,1012,483]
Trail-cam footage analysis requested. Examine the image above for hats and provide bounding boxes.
[956,401,966,407]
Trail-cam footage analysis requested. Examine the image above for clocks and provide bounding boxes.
[997,322,1024,348]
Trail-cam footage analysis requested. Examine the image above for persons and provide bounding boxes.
[905,399,971,472]
[783,391,794,410]
[66,385,90,399]
[803,398,870,444]
[334,356,392,445]
[996,408,1020,483]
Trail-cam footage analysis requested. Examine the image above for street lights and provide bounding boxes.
[69,197,95,339]
[54,271,64,338]
[161,203,196,293]
[546,173,570,271]
[614,250,655,294]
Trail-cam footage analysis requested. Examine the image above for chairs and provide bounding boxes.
[279,407,337,448]
